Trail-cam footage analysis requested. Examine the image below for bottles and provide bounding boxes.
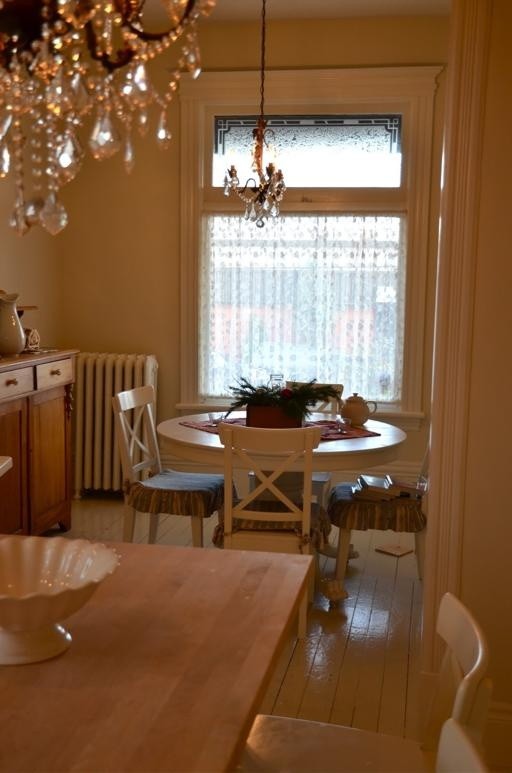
[267,375,286,391]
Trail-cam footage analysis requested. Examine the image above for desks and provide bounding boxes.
[154,411,407,507]
[1,534,313,773]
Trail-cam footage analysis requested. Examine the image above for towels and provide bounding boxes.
[179,417,381,440]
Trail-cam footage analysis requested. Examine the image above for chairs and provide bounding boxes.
[238,591,488,772]
[111,384,240,547]
[435,718,485,773]
[328,408,430,592]
[248,379,344,508]
[211,421,333,640]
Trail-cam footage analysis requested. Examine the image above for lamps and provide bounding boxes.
[223,1,288,228]
[1,0,217,235]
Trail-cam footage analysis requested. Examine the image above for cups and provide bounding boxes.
[337,418,351,434]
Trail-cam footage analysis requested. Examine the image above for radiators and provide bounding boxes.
[73,350,160,500]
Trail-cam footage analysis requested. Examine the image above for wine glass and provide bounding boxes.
[0,534,122,667]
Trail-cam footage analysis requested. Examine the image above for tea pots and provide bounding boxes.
[337,393,377,426]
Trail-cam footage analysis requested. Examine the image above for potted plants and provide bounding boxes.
[223,374,340,429]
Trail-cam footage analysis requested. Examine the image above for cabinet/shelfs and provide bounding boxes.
[0,348,82,536]
